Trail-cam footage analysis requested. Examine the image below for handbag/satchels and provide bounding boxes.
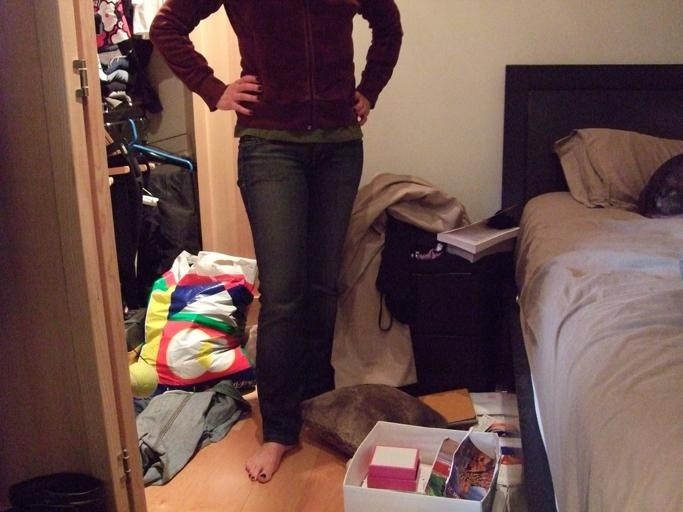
[137,249,258,387]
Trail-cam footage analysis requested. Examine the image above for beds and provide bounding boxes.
[500,62,680,510]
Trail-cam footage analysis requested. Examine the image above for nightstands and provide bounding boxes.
[409,239,504,393]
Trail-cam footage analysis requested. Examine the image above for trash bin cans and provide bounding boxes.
[8,472,108,512]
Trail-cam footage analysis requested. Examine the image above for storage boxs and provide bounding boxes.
[342,419,502,507]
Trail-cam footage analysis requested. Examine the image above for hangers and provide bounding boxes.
[124,118,198,175]
[137,119,195,171]
[99,122,149,176]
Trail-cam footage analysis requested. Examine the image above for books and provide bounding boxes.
[438,205,521,264]
[414,388,478,427]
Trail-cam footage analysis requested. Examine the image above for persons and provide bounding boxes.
[149,1,404,483]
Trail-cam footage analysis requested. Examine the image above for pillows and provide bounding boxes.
[300,384,448,458]
[558,128,683,212]
